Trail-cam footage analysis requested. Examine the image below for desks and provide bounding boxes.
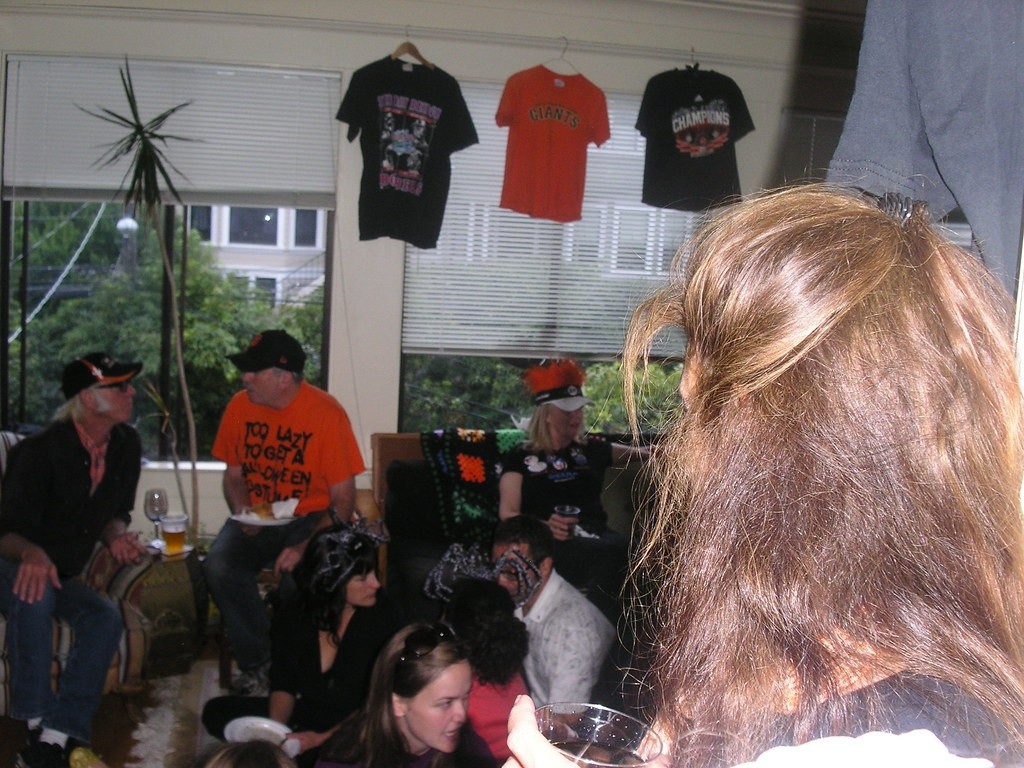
[138,544,208,681]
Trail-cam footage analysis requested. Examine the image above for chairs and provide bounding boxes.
[217,570,284,689]
[0,430,162,726]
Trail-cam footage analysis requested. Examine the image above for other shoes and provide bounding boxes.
[14,728,71,768]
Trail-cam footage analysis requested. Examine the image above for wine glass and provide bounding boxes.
[143,489,169,549]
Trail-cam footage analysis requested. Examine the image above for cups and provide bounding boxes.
[534,702,663,767]
[159,513,188,555]
[554,505,580,539]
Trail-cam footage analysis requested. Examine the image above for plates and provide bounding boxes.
[228,516,297,526]
[223,715,292,744]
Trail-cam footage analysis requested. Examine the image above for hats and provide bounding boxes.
[63,352,143,401]
[225,328,306,374]
[533,385,596,412]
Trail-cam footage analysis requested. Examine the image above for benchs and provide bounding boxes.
[370,432,667,682]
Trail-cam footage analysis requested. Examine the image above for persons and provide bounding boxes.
[201,524,404,768]
[309,618,501,768]
[502,170,1023,768]
[492,515,617,738]
[443,575,528,765]
[203,329,368,697]
[205,738,299,768]
[0,352,148,768]
[498,360,659,609]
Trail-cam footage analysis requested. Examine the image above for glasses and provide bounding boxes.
[93,379,131,393]
[393,622,460,682]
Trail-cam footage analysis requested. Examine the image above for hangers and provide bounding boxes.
[679,46,709,76]
[391,23,434,70]
[543,36,581,75]
